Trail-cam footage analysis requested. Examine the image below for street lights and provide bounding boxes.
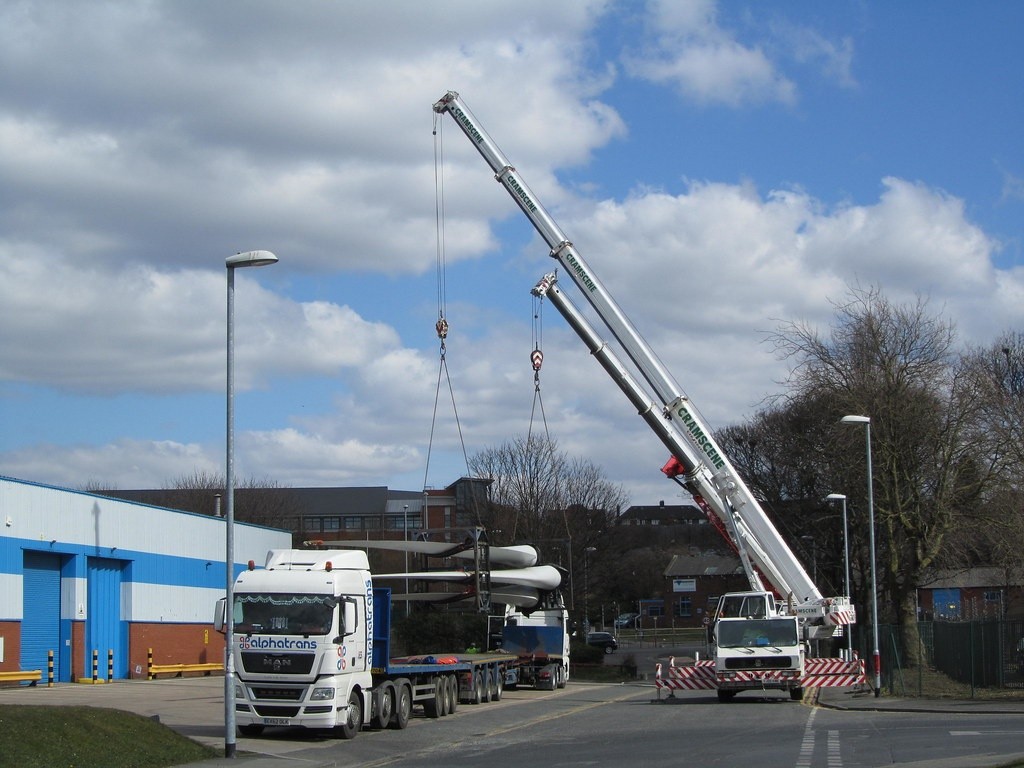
[839,411,887,696]
[828,491,852,653]
[220,247,279,756]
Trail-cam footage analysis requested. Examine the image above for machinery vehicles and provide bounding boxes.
[434,88,857,705]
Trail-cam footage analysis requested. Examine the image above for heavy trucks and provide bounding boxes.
[213,546,576,739]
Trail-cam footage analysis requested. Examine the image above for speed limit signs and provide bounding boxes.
[703,617,710,625]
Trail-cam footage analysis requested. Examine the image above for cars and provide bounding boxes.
[615,612,637,629]
[586,631,619,654]
